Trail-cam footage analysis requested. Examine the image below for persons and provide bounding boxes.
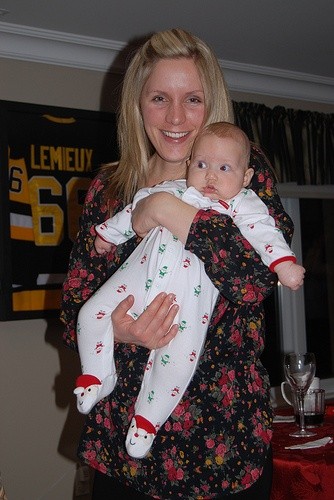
[73,120,306,460]
[57,26,296,500]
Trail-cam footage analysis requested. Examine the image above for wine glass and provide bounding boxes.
[283,352,317,437]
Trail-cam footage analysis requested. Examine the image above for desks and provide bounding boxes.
[271,399,334,500]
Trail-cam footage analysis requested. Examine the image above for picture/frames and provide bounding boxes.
[0,99,120,321]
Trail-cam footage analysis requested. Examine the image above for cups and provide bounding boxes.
[293,389,326,429]
[281,377,320,407]
